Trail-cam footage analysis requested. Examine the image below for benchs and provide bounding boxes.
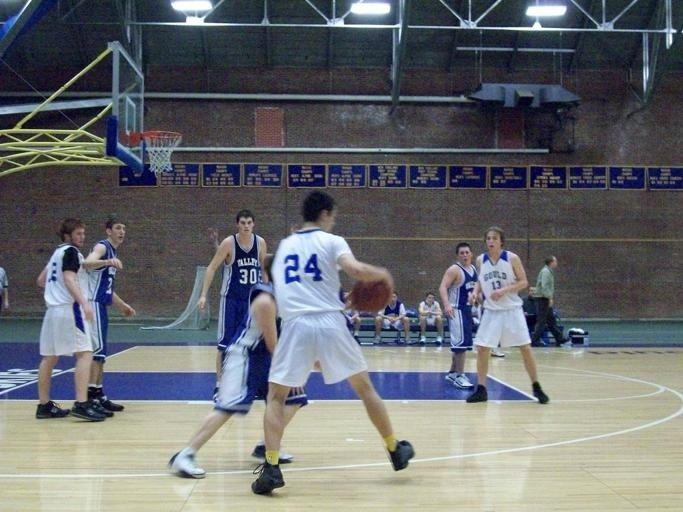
[527,309,564,346]
[358,309,450,348]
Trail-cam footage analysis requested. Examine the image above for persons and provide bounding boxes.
[471,255,568,357]
[343,294,443,345]
[467,227,549,404]
[438,242,479,389]
[36,218,106,422]
[167,255,308,480]
[81,218,136,416]
[0,267,9,315]
[197,209,269,402]
[250,188,414,494]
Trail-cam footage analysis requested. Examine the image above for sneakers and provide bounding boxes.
[69,401,106,423]
[34,401,70,420]
[212,386,219,403]
[532,381,548,404]
[168,452,205,480]
[444,371,469,382]
[251,460,284,495]
[351,335,571,346]
[386,440,415,472]
[88,398,114,418]
[453,374,473,390]
[252,441,292,460]
[95,395,124,412]
[466,384,487,403]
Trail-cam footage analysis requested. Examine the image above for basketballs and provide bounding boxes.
[352,278,391,312]
